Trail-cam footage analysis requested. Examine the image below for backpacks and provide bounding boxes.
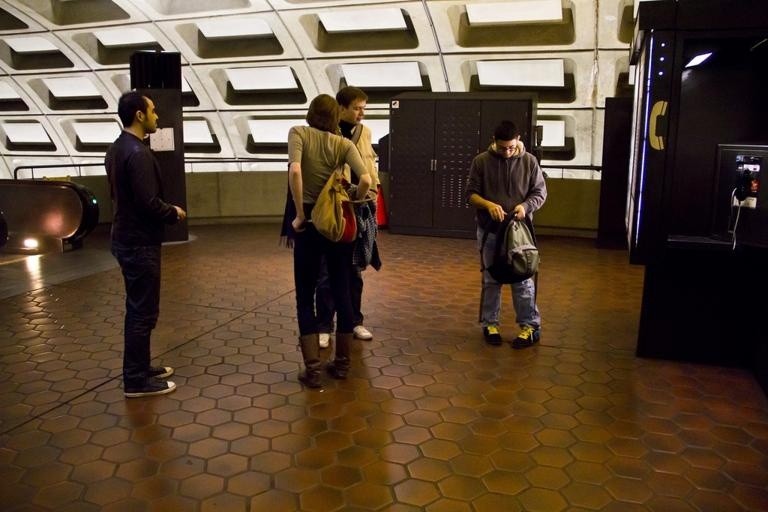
[479,210,541,284]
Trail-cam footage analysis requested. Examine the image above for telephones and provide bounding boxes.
[736,171,751,201]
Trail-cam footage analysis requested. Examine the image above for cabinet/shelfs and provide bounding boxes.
[386,89,539,241]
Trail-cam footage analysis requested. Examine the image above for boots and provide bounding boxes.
[297,332,323,389]
[325,328,354,380]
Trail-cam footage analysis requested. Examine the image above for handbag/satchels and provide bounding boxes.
[311,166,358,243]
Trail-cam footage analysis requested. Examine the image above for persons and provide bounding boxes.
[316,84,387,352]
[464,117,548,350]
[372,127,390,212]
[103,86,188,402]
[280,93,371,392]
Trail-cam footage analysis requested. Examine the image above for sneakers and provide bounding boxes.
[513,325,541,347]
[149,365,173,380]
[318,332,331,349]
[482,325,505,346]
[352,323,373,342]
[124,377,177,398]
